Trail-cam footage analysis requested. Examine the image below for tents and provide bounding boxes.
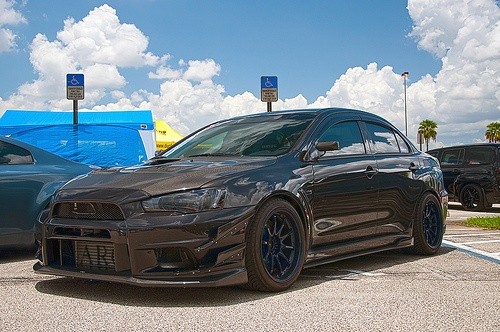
[154,120,213,152]
[0,110,156,169]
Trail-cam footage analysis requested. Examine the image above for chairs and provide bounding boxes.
[242,133,301,150]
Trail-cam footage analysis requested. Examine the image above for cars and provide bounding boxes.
[0,136,114,259]
[33,106,450,293]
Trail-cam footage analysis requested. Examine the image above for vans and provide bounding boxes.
[425,144,500,212]
